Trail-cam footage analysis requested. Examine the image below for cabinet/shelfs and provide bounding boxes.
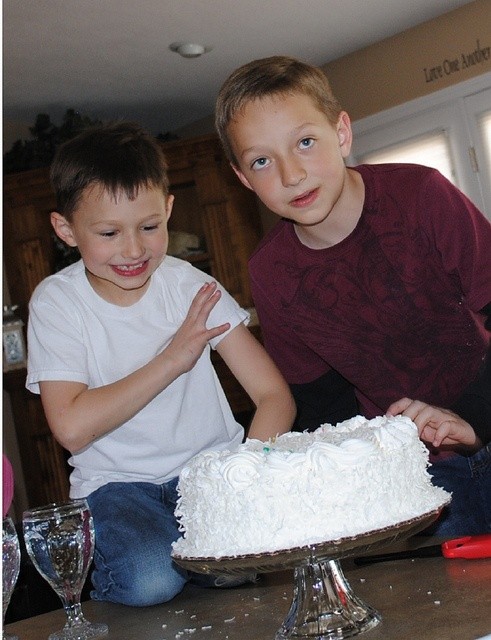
[0,139,258,510]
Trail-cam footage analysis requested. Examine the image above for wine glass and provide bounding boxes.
[21,502,109,636]
[2,516,20,638]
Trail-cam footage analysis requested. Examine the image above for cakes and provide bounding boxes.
[170,414,454,559]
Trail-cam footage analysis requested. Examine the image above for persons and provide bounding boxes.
[24,123,298,607]
[214,55,491,537]
